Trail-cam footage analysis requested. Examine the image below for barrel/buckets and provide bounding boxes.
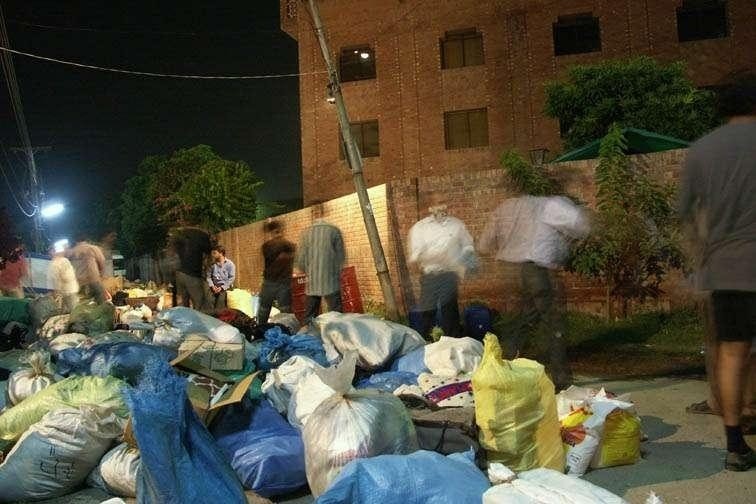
[409,305,439,333]
[464,306,502,340]
[290,272,324,325]
[339,266,364,313]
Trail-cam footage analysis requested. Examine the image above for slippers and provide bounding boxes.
[683,399,722,417]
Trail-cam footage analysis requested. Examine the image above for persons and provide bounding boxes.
[403,190,477,339]
[249,219,297,324]
[67,232,109,304]
[670,62,755,473]
[291,202,348,316]
[476,181,597,392]
[162,211,214,312]
[1,239,31,302]
[42,243,81,310]
[683,250,756,437]
[205,243,236,311]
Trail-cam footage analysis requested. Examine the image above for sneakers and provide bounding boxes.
[724,445,756,472]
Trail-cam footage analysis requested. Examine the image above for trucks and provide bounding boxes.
[113,250,127,279]
